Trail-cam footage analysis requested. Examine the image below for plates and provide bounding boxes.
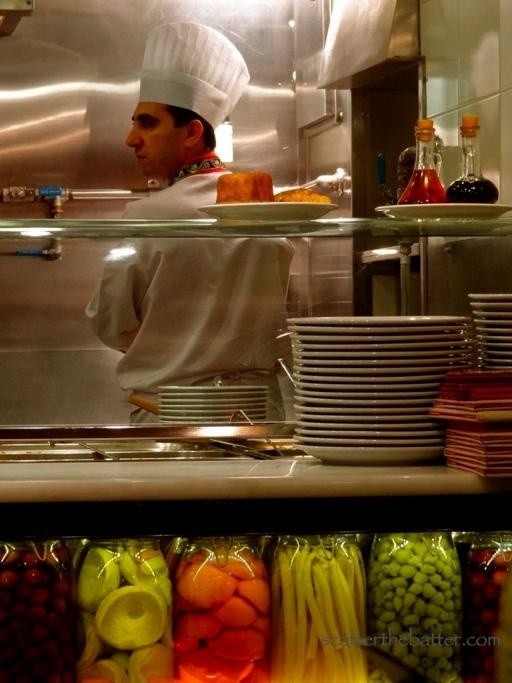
[467,290,512,369]
[375,200,512,220]
[195,200,340,221]
[156,383,270,427]
[285,314,473,464]
[430,369,511,479]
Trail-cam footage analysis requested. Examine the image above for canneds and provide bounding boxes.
[0,531,512,683]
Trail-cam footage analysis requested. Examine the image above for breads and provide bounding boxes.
[216,172,332,203]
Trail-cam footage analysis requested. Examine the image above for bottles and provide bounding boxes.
[445,114,501,205]
[164,532,275,683]
[393,118,445,205]
[76,536,177,683]
[367,531,465,683]
[460,530,512,683]
[261,534,368,683]
[0,535,83,683]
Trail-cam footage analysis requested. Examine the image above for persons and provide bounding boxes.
[84,101,299,427]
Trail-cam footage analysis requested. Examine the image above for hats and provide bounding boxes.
[138,22,253,129]
